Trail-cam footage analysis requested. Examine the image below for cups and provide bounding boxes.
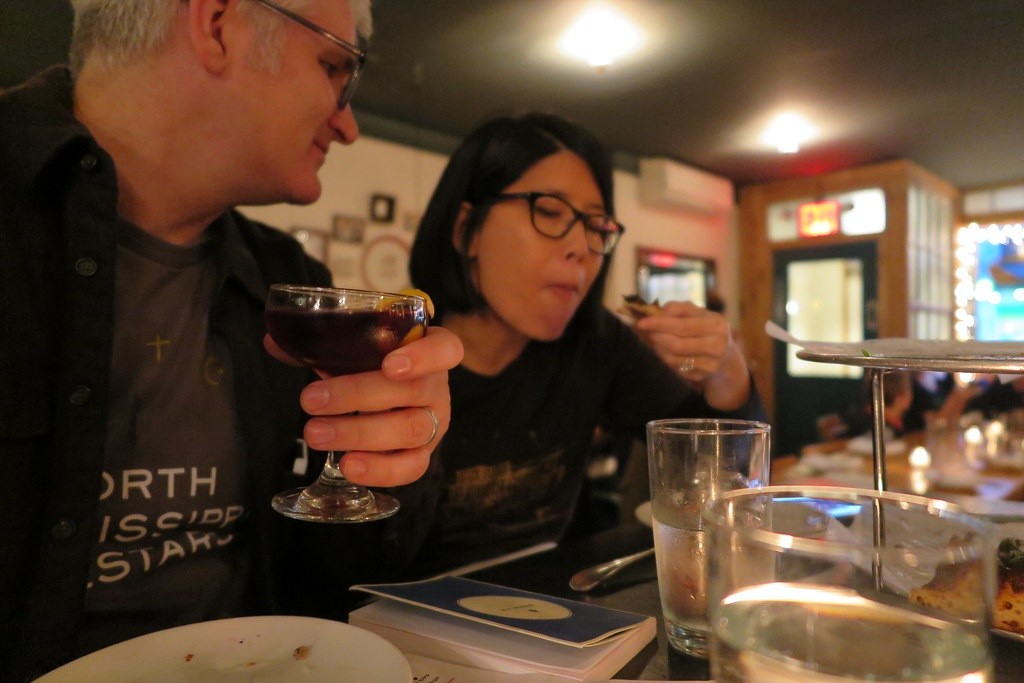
[650,418,771,660]
[707,490,994,683]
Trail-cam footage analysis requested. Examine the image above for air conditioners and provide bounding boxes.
[641,158,735,225]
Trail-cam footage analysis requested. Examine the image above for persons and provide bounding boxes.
[766,369,1024,461]
[388,111,768,583]
[1,2,464,683]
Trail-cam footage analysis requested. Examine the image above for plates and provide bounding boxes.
[638,495,991,573]
[32,616,413,683]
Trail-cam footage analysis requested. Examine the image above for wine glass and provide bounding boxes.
[263,285,425,524]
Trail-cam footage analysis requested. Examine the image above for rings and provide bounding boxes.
[680,356,694,377]
[421,408,438,446]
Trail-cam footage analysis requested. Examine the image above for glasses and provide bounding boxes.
[257,0,369,111]
[489,191,625,255]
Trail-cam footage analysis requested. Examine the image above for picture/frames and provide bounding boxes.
[292,227,330,267]
[334,214,365,244]
[370,194,395,221]
